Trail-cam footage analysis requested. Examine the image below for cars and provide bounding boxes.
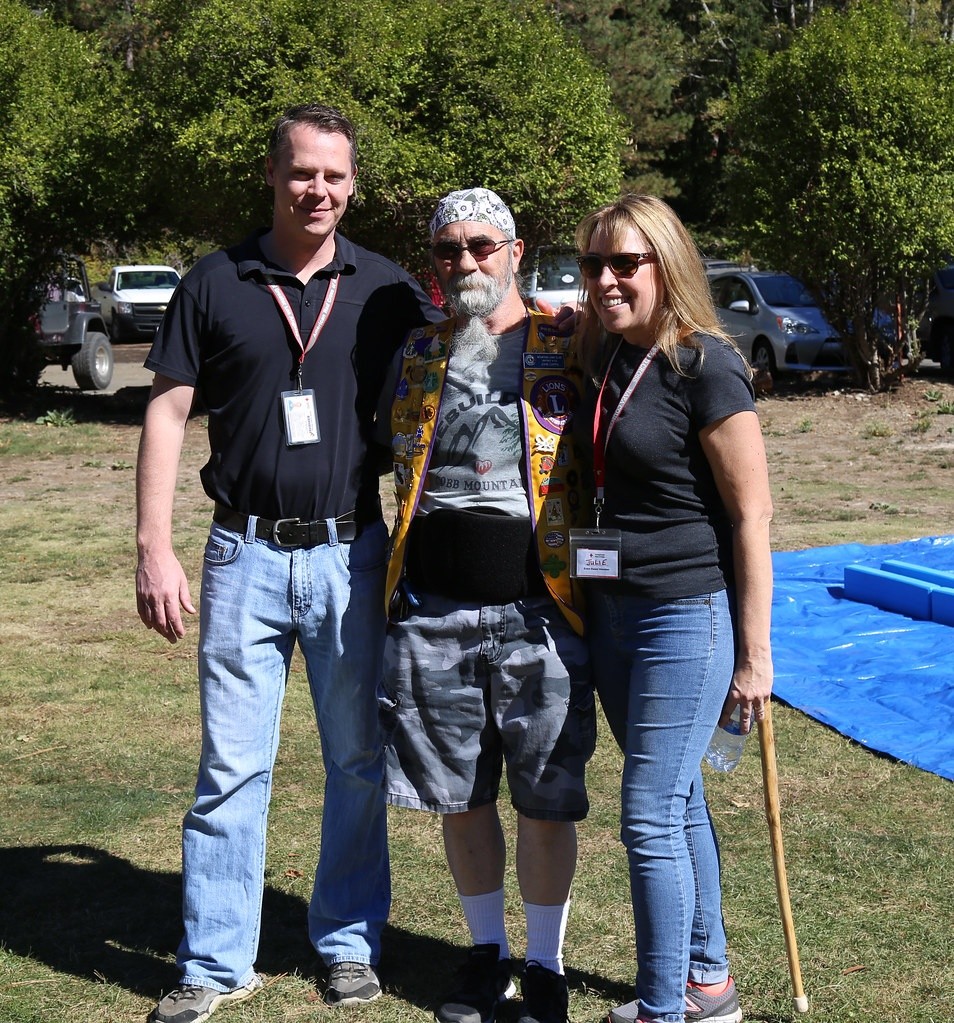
[703,260,759,282]
[913,267,954,381]
[710,270,865,387]
[521,243,591,310]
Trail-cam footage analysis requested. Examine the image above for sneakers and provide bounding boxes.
[434,943,516,1023]
[512,959,569,1023]
[326,962,382,1006]
[608,977,742,1023]
[148,978,256,1023]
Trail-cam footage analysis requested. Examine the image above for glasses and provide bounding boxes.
[576,253,658,280]
[431,237,515,261]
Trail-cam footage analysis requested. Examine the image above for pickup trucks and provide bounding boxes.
[88,261,183,344]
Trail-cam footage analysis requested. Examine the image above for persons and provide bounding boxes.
[572,194,774,1023]
[134,103,583,1023]
[375,187,599,1023]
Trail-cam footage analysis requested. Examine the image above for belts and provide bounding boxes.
[214,503,383,548]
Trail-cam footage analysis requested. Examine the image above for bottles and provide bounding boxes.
[704,703,755,771]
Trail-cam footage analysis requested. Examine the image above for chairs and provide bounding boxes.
[154,275,165,283]
[121,274,132,289]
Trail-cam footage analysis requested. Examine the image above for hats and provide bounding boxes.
[429,187,516,242]
